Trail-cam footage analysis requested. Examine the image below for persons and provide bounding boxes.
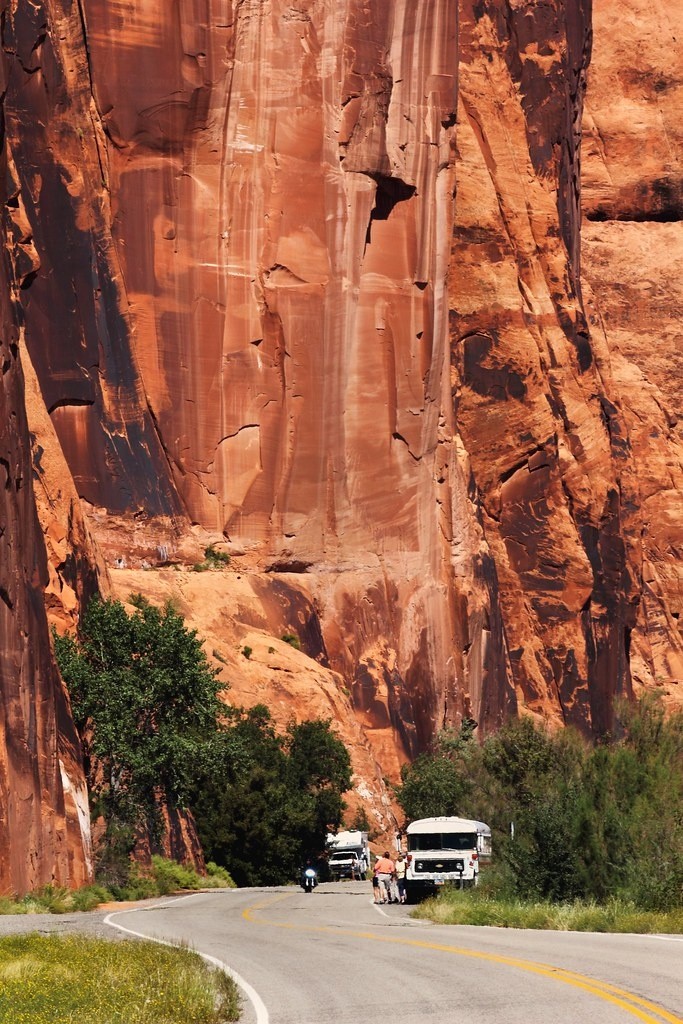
[301,859,318,887]
[372,851,408,905]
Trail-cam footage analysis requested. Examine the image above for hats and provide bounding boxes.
[376,855,383,859]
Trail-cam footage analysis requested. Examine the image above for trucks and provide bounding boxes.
[325,830,370,882]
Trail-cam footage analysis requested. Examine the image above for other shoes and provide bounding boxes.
[374,897,406,904]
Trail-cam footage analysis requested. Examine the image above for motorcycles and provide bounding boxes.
[300,865,319,893]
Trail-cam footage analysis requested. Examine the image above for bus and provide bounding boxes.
[405,816,492,905]
